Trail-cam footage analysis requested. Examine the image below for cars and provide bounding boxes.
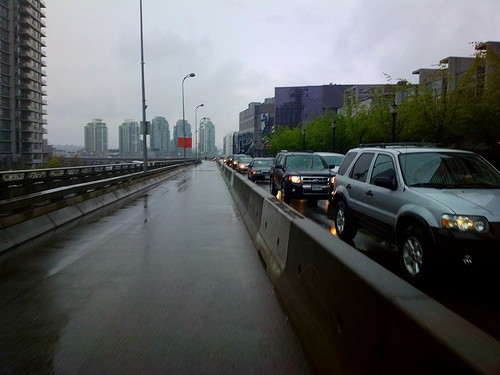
[214,156,220,161]
[237,158,253,174]
[248,157,275,182]
[223,154,250,169]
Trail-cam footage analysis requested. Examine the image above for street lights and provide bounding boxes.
[331,119,337,153]
[302,129,306,150]
[390,100,398,144]
[182,72,196,163]
[195,103,206,161]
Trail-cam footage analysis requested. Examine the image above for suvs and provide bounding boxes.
[313,152,346,173]
[327,145,500,280]
[270,150,336,203]
